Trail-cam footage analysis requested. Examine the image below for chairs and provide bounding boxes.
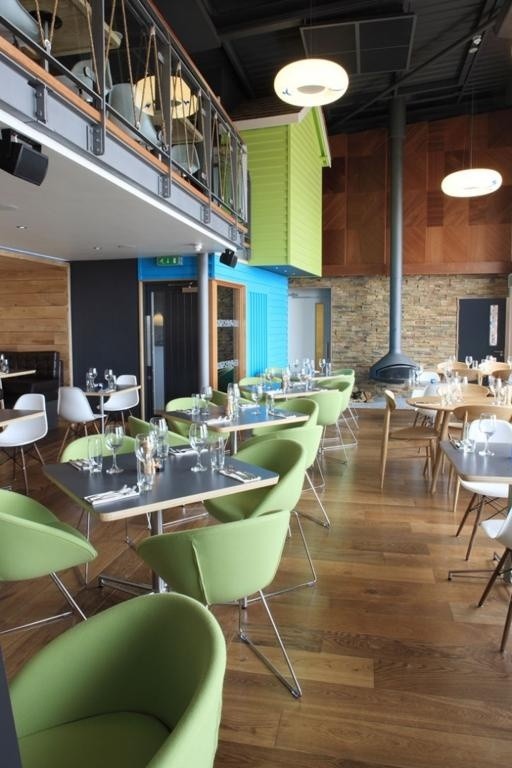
[1,486,97,636]
[380,389,443,492]
[407,353,510,510]
[8,592,226,768]
[0,0,200,182]
[1,349,357,545]
[457,418,511,560]
[202,437,319,608]
[134,509,303,699]
[477,508,511,654]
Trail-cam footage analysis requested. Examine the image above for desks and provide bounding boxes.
[40,448,279,594]
[438,440,510,581]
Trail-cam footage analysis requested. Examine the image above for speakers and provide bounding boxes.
[220,253,237,268]
[0,140,49,187]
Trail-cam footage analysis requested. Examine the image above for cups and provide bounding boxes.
[463,423,477,453]
[85,368,117,391]
[441,353,512,406]
[260,358,332,393]
[191,383,276,416]
[134,416,170,494]
[209,434,225,471]
[0,353,10,374]
[87,437,104,473]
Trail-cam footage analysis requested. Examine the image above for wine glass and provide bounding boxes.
[479,413,496,456]
[189,423,208,473]
[104,425,125,473]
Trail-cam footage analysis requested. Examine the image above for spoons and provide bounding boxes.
[86,486,136,503]
[170,448,198,454]
[222,464,257,481]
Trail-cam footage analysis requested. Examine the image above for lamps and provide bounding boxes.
[274,0,350,108]
[439,85,503,200]
